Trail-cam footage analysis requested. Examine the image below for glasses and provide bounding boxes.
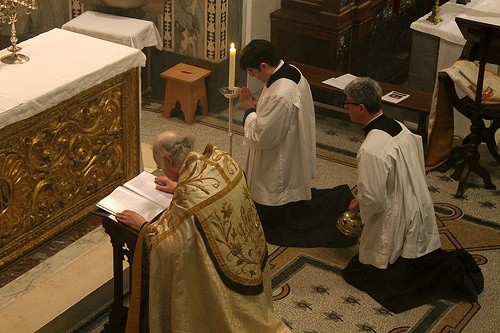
[344,100,362,109]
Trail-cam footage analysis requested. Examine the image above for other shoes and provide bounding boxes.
[453,267,480,302]
[456,248,484,294]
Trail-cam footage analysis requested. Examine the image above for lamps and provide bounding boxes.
[0,0,38,64]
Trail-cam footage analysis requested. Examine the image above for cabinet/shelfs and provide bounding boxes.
[270,0,412,83]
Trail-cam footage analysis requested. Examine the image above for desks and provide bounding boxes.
[288,62,432,137]
[409,0,500,149]
[63,11,156,101]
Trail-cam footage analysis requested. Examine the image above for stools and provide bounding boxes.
[161,63,211,123]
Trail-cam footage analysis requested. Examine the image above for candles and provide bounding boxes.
[228,42,236,90]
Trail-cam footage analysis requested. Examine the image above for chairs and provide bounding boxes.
[438,16,500,199]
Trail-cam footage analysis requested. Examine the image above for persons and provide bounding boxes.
[343,76,484,303]
[238,39,352,246]
[115,127,291,333]
